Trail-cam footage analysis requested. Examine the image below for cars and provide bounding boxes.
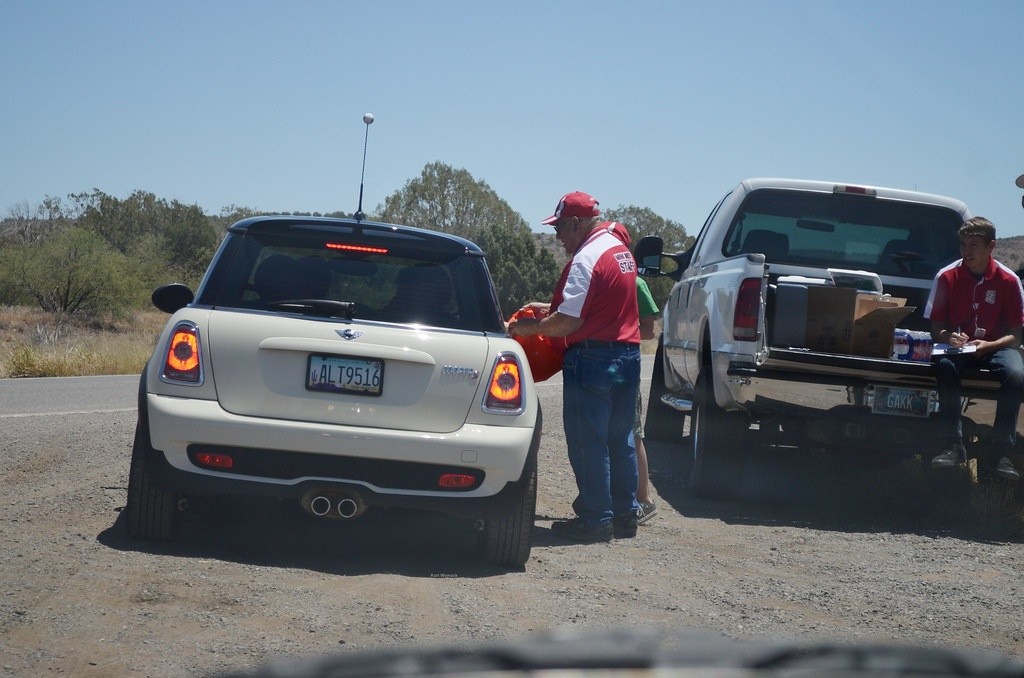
[128,214,546,570]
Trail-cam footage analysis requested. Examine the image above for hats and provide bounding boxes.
[541,191,600,224]
[603,219,631,247]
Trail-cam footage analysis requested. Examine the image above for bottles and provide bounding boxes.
[895,328,932,363]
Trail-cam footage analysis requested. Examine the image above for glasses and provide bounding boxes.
[554,216,583,234]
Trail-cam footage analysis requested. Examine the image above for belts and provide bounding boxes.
[567,341,633,349]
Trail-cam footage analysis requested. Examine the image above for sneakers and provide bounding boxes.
[614,512,638,539]
[932,443,969,469]
[988,443,1019,479]
[552,515,613,543]
[638,501,657,524]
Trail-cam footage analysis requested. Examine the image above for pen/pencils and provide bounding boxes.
[958,326,961,335]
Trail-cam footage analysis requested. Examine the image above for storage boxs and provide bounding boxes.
[771,269,917,360]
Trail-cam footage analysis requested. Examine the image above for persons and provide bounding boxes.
[507,191,642,541]
[924,216,1024,481]
[602,221,656,523]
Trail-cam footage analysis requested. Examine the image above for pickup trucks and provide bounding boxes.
[631,176,1024,517]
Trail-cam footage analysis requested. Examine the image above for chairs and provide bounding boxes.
[377,261,459,329]
[741,230,790,263]
[882,240,925,278]
[241,254,337,322]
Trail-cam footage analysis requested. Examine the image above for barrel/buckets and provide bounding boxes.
[505,308,565,382]
